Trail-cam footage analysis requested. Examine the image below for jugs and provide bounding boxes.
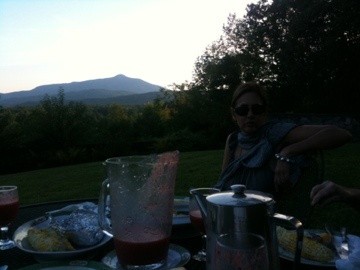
[98,151,181,269]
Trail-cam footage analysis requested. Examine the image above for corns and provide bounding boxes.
[274,226,334,262]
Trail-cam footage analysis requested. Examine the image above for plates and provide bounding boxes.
[102,243,191,270]
[16,261,112,270]
[14,213,113,256]
[170,196,199,225]
[278,229,360,267]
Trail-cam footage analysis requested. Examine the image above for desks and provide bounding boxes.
[0,199,339,270]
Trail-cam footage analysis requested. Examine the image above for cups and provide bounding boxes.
[0,186,19,250]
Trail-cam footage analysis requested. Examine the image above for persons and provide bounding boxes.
[217,83,353,205]
[309,180,360,210]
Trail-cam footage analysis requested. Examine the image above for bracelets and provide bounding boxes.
[274,153,294,164]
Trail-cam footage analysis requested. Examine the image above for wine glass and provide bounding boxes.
[189,187,221,262]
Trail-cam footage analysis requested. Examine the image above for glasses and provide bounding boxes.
[234,107,265,116]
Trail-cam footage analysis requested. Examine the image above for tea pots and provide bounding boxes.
[189,184,303,270]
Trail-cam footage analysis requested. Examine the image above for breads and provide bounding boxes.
[27,213,103,252]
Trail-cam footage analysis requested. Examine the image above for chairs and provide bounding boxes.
[278,149,325,230]
